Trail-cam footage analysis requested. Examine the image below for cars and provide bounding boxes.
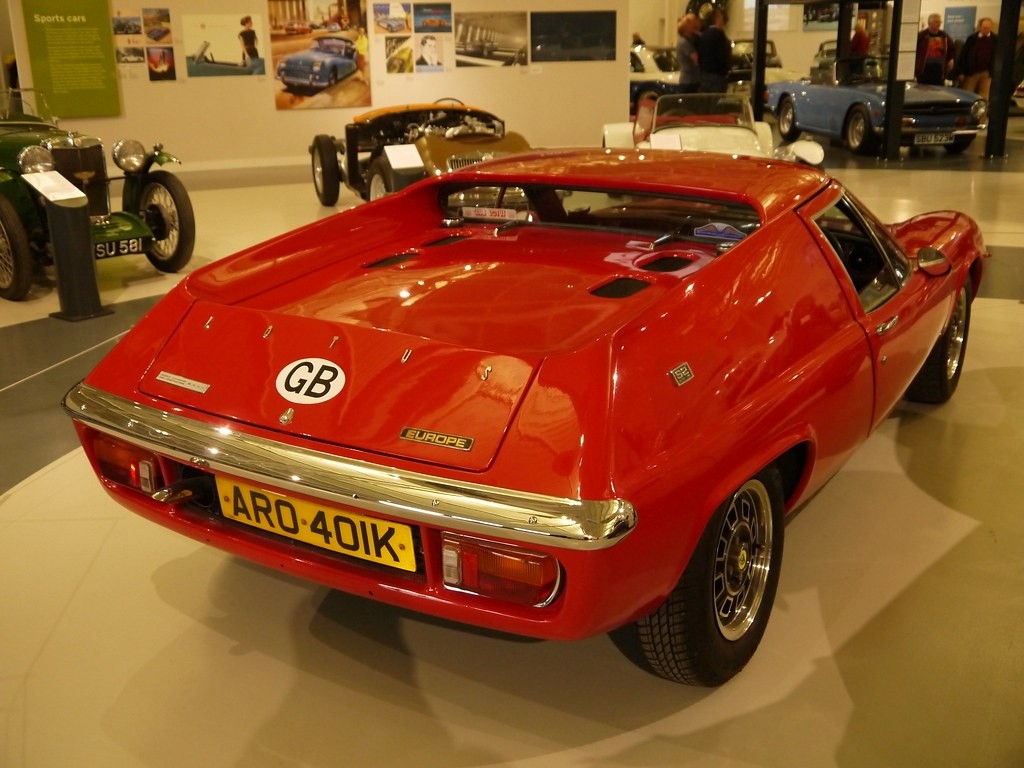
[274,35,358,89]
[0,87,197,301]
[729,37,783,81]
[309,97,573,215]
[327,23,341,32]
[762,58,989,158]
[62,148,989,691]
[600,91,825,173]
[630,44,682,115]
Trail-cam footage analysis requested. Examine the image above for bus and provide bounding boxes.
[285,21,312,35]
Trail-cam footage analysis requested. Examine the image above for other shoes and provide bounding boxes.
[977,123,985,129]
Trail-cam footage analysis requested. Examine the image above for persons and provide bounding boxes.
[915,13,954,86]
[957,17,997,128]
[351,27,367,84]
[676,0,732,102]
[849,23,870,74]
[633,33,645,44]
[239,15,259,67]
